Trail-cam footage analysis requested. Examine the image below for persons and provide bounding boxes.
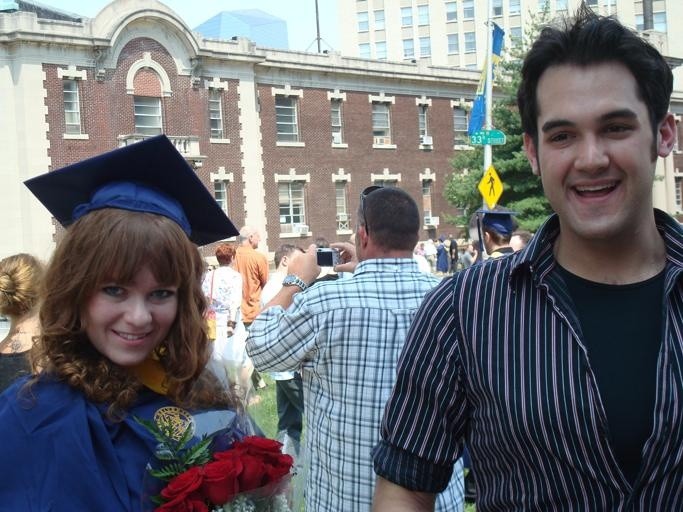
[245,183,465,510]
[369,2,683,511]
[414,210,533,277]
[202,226,338,439]
[1,253,52,390]
[1,207,298,510]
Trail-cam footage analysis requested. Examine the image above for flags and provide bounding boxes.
[468,23,505,135]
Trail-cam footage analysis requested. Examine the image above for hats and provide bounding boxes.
[23,134,240,246]
[471,211,521,251]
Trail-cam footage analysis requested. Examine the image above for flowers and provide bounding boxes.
[131,415,295,512]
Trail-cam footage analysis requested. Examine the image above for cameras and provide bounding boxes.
[316,248,340,268]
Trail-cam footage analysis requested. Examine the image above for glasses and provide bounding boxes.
[360,185,384,235]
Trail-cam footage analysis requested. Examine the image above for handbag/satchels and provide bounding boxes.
[200,308,216,340]
